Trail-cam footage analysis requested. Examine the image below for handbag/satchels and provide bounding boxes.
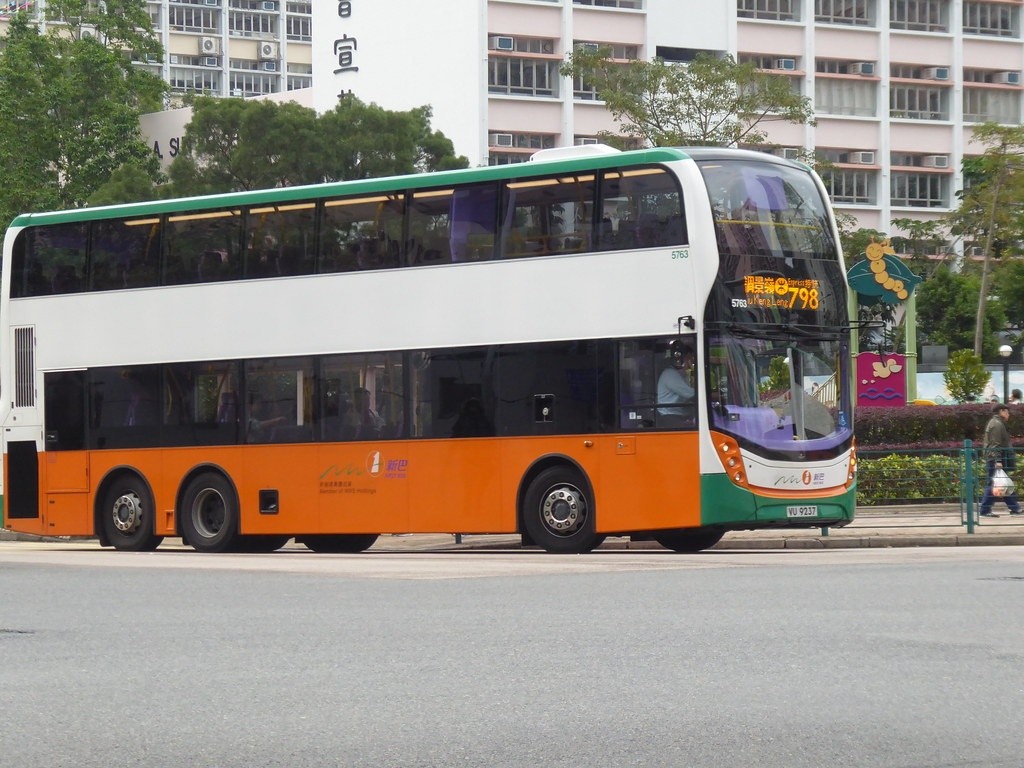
[992,468,1015,497]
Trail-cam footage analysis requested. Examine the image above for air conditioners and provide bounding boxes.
[74,27,98,40]
[488,133,513,147]
[256,2,274,10]
[488,36,513,51]
[203,0,217,6]
[256,41,277,72]
[770,148,948,168]
[574,137,597,146]
[198,37,218,67]
[573,43,598,57]
[773,58,1022,85]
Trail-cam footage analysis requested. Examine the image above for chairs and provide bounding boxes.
[123,388,480,442]
[27,211,685,295]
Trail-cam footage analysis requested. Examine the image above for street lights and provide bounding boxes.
[998,346,1012,403]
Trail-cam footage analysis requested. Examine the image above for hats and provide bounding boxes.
[992,403,1009,412]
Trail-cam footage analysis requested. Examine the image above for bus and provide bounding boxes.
[0,144,887,555]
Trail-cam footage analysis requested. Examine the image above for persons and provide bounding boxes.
[990,395,999,403]
[222,416,288,432]
[978,403,1024,517]
[656,344,696,426]
[336,387,382,439]
[1009,389,1023,405]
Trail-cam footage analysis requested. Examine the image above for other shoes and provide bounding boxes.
[1009,507,1024,515]
[980,512,999,518]
